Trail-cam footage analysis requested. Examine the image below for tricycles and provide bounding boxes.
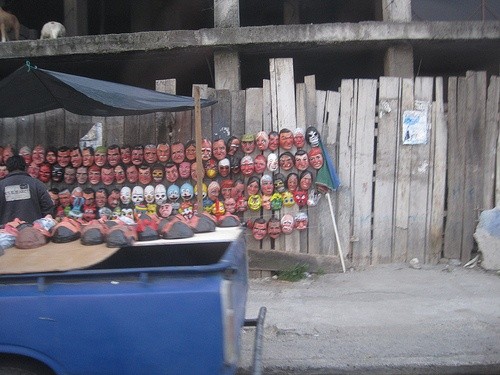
[0,222,267,375]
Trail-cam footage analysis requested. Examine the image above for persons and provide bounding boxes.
[0,155,57,225]
